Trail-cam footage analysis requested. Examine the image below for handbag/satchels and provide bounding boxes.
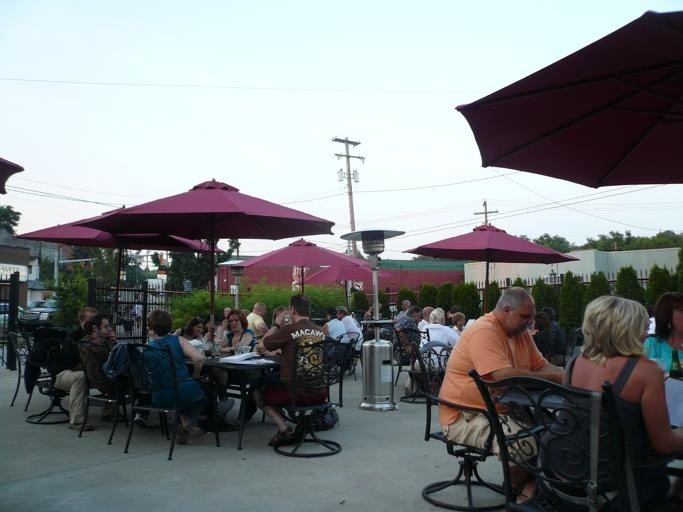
[199,373,220,391]
[310,407,339,430]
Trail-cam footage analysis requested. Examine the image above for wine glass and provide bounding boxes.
[242,344,251,354]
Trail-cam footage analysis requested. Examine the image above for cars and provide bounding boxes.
[17,300,70,331]
[0,302,24,333]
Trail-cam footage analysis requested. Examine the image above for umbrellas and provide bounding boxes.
[0,157,25,183]
[233,234,387,288]
[399,221,583,321]
[451,10,681,188]
[63,177,334,433]
[15,202,228,343]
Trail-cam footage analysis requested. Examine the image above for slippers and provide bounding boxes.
[509,500,545,512]
[503,482,545,501]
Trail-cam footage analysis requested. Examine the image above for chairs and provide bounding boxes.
[533,324,583,371]
[468,366,634,510]
[319,313,454,403]
[273,340,349,457]
[407,340,508,511]
[8,317,280,461]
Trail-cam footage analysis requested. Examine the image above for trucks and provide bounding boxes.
[110,280,168,314]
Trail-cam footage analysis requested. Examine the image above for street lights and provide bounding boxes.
[337,167,361,258]
[230,265,243,311]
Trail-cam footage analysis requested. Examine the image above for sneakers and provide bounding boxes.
[69,385,302,446]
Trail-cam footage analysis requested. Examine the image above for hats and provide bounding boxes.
[327,308,336,314]
[336,306,348,313]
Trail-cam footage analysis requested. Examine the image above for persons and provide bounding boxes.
[434,281,566,511]
[0,279,682,510]
[561,294,681,511]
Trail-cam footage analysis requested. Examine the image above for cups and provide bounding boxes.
[204,342,213,360]
[233,341,243,357]
[214,340,224,358]
